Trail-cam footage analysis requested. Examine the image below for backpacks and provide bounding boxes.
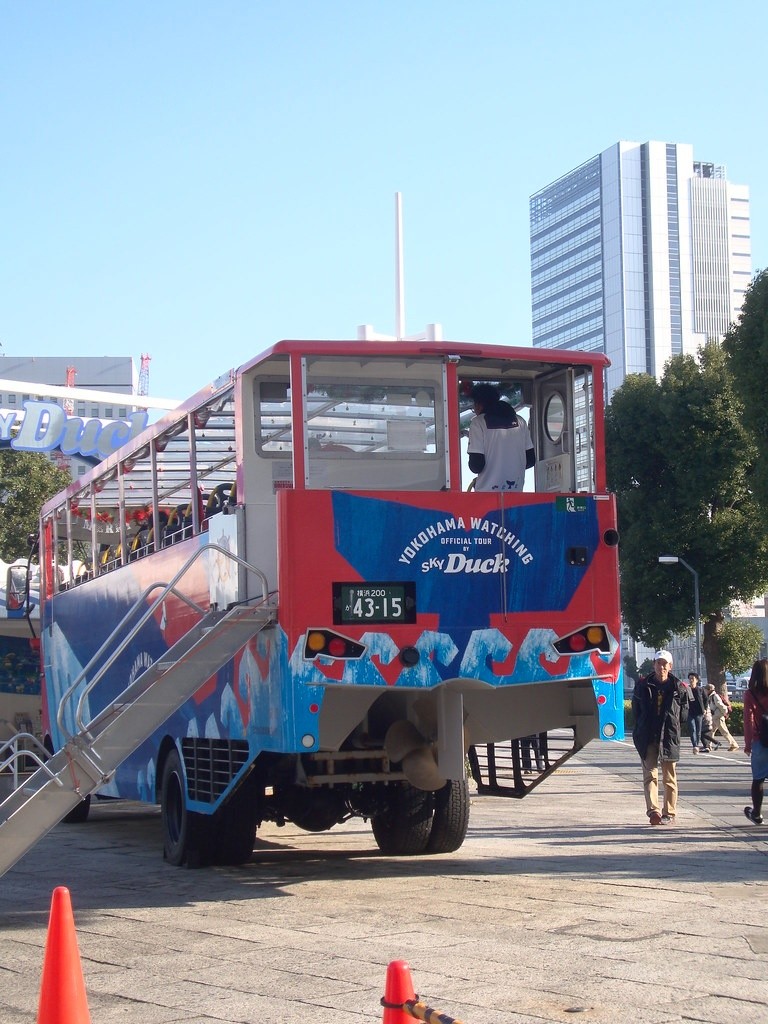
[748,688,768,749]
[708,692,732,714]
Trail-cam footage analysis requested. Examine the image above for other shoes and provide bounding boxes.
[650,812,662,825]
[744,807,764,826]
[693,747,699,755]
[714,742,722,751]
[660,815,674,825]
[727,747,739,751]
[700,748,709,752]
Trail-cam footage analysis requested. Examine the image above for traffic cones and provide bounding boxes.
[380,960,423,1024]
[32,886,94,1024]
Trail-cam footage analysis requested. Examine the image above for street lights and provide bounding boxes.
[657,556,702,686]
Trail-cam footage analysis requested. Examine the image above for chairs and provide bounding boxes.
[76,480,241,584]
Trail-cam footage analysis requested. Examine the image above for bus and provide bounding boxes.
[5,339,625,867]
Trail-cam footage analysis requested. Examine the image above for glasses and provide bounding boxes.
[471,403,476,413]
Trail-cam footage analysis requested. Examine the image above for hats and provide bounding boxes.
[703,684,715,691]
[653,650,673,664]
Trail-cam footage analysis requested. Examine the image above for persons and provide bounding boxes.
[743,658,768,825]
[146,510,170,552]
[686,670,739,756]
[467,383,535,492]
[630,649,689,825]
